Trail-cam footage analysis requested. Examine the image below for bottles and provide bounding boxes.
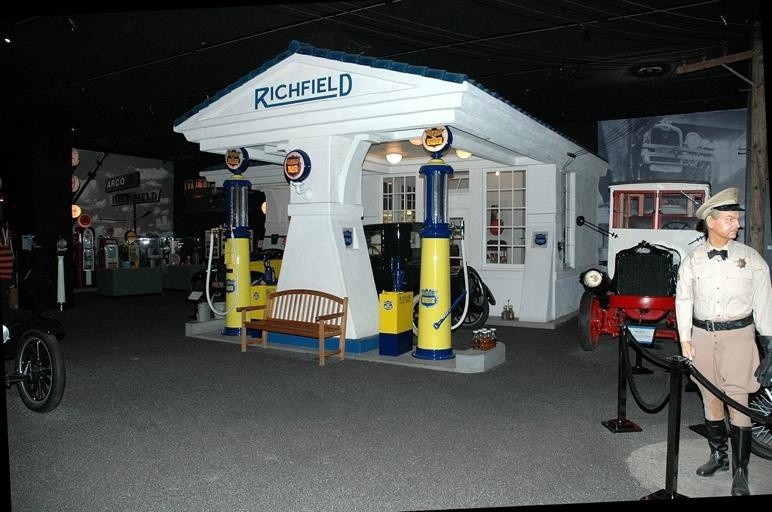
[472,328,497,351]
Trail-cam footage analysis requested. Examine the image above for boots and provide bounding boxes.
[729,422,751,496]
[696,418,729,476]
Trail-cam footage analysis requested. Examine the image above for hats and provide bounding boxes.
[696,188,746,220]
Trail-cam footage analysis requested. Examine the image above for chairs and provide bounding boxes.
[625,215,699,229]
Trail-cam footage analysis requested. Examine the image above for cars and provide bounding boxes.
[362,221,496,337]
[579,180,712,352]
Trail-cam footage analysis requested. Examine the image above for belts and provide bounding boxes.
[692,315,753,332]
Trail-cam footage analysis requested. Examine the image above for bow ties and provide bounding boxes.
[708,249,728,260]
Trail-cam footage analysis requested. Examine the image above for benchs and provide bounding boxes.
[233,287,350,367]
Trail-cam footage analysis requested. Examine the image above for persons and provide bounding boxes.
[675,186,772,496]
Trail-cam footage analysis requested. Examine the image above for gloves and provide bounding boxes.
[755,334,772,387]
